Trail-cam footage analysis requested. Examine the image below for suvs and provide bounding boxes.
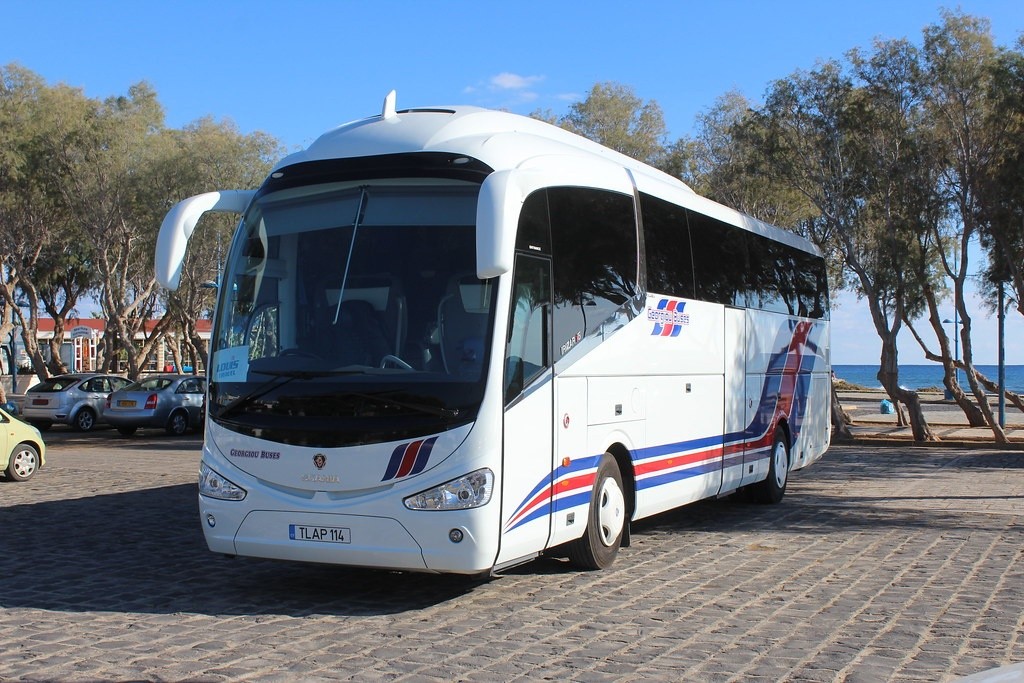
[22,373,135,432]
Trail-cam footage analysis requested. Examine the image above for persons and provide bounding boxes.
[831,370,835,380]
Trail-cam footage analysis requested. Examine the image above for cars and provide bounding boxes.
[0,407,46,481]
[102,374,212,437]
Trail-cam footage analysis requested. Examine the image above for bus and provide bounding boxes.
[153,90,833,582]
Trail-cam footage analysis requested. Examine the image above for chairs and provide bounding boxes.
[439,268,490,378]
[94,381,104,391]
[311,298,399,371]
